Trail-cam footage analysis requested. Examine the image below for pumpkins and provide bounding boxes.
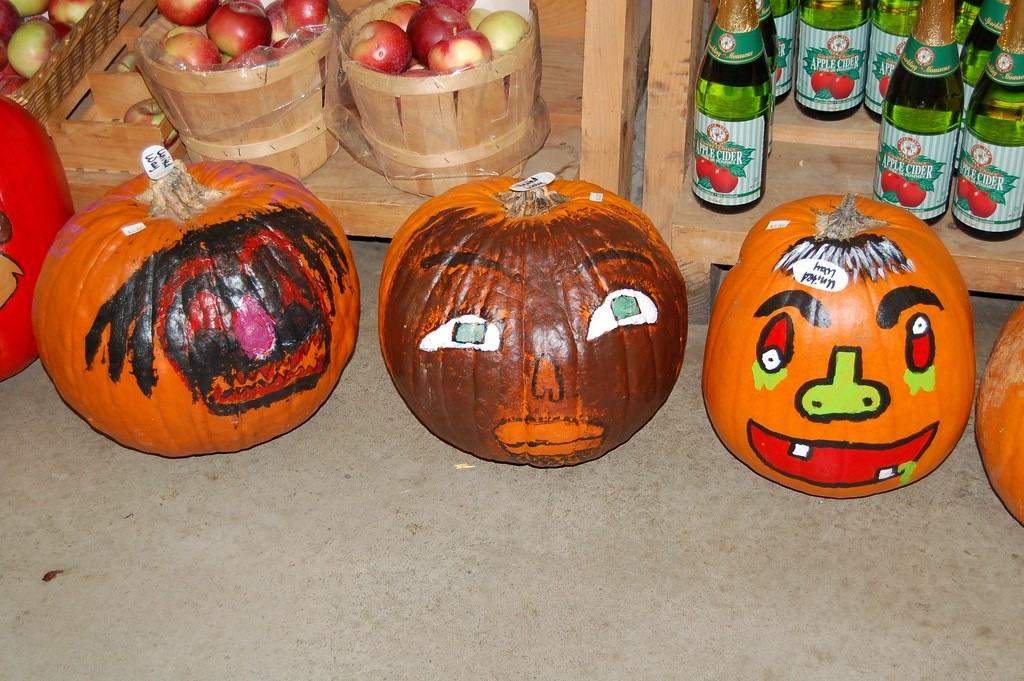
[33,143,1024,527]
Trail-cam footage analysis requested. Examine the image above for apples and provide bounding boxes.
[1,1,531,132]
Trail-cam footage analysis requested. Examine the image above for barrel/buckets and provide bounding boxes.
[340,0,538,196]
[136,6,340,180]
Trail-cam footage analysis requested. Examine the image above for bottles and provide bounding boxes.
[705,0,780,156]
[957,0,982,61]
[952,1,1024,246]
[764,0,799,106]
[690,1,771,215]
[871,1,965,225]
[795,0,868,121]
[952,0,1012,177]
[863,0,921,124]
